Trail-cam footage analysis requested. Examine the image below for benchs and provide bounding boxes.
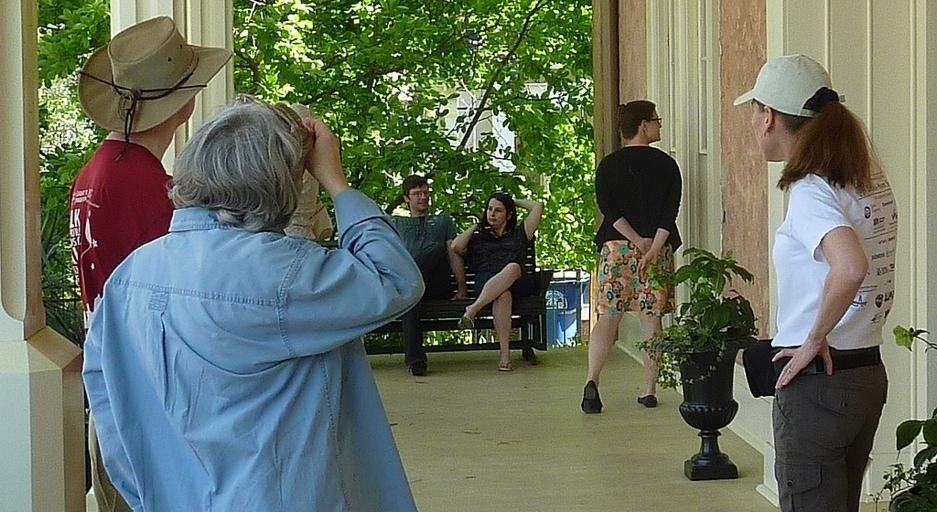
[312,239,554,366]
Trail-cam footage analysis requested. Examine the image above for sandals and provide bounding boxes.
[456,314,475,330]
[497,359,514,373]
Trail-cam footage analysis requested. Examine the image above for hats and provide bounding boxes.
[78,16,234,134]
[733,53,847,117]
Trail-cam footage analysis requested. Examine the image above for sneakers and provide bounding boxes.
[410,360,428,375]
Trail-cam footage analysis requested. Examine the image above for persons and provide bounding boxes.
[581,100,682,412]
[68,15,233,340]
[733,55,899,512]
[81,96,426,511]
[389,174,469,376]
[450,193,543,372]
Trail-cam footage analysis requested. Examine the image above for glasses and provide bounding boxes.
[649,116,666,125]
[494,190,509,196]
[403,190,429,196]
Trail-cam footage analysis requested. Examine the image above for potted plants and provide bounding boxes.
[635,248,759,480]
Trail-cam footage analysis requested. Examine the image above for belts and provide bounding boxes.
[776,346,881,376]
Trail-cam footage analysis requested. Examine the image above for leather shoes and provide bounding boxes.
[636,394,659,407]
[579,380,603,415]
